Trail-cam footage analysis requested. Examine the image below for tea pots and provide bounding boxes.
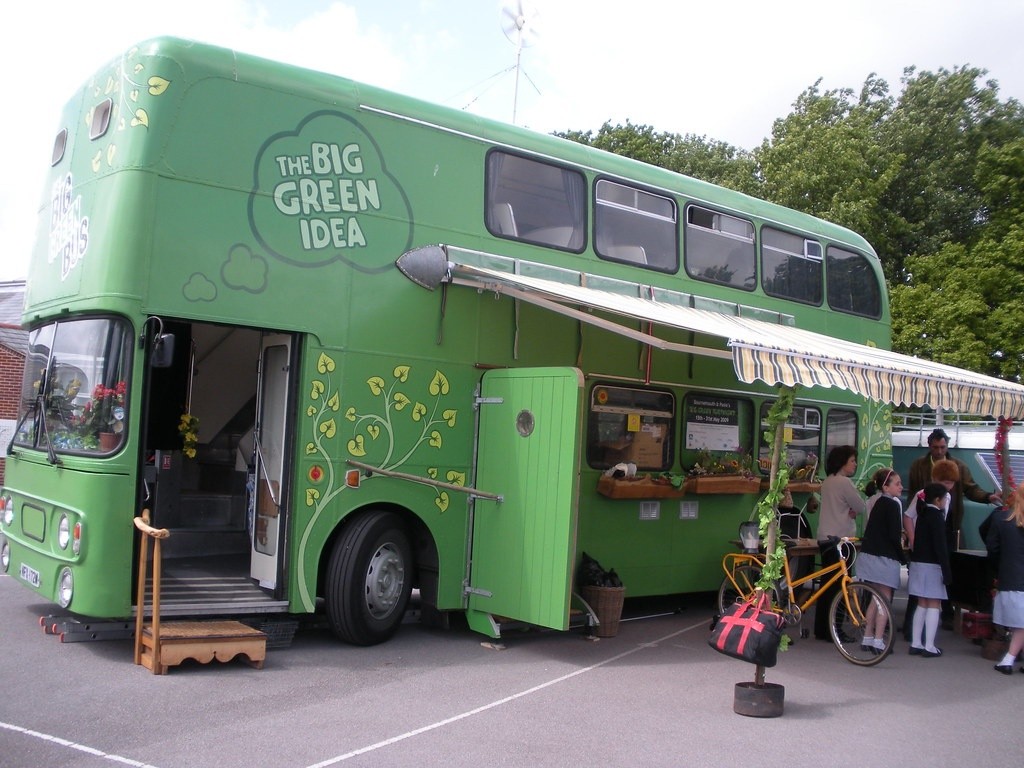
[739,521,762,554]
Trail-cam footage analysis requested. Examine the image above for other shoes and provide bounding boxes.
[994,664,1012,675]
[861,644,873,652]
[815,631,826,640]
[902,621,913,642]
[871,646,893,655]
[1019,666,1024,673]
[826,630,857,643]
[941,611,954,630]
[922,647,943,657]
[909,646,924,655]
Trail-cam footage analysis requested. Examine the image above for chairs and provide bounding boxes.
[491,203,648,267]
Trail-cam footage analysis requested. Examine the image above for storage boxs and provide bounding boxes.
[962,612,993,639]
[596,423,667,469]
[240,616,300,647]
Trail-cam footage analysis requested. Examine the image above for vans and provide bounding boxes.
[890,411,1024,556]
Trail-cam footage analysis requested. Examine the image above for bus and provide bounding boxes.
[0,36,896,651]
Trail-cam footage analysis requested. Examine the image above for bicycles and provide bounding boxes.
[719,536,895,666]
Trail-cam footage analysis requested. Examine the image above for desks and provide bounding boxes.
[952,548,991,644]
[732,536,863,601]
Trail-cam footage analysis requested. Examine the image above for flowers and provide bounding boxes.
[178,414,198,460]
[22,368,128,450]
[685,446,761,480]
[993,416,1019,511]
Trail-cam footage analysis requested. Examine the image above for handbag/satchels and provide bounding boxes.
[708,593,785,667]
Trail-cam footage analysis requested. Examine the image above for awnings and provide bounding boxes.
[447,259,1024,421]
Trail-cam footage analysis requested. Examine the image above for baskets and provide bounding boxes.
[578,585,626,637]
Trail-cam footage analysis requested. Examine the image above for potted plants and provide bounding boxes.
[598,468,687,499]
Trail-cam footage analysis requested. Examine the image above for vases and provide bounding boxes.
[99,431,119,451]
[687,474,762,494]
[764,480,822,492]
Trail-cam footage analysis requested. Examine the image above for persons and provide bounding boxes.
[978,479,1024,675]
[814,445,866,643]
[854,429,1004,659]
